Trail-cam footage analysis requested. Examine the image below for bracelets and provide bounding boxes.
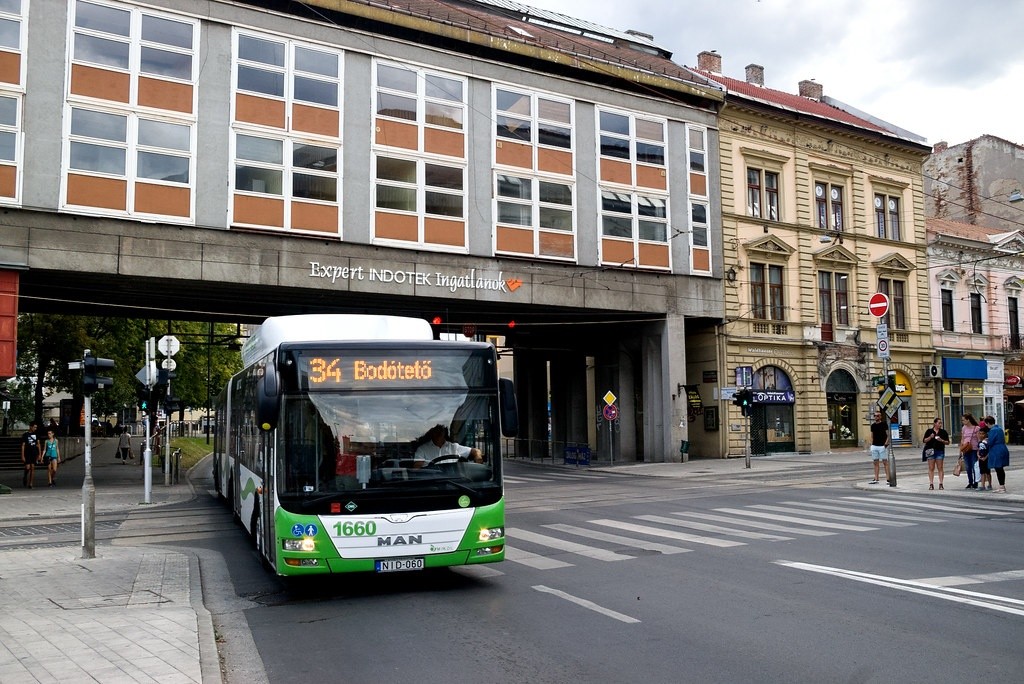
[940,439,944,443]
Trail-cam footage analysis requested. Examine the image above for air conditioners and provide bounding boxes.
[925,365,941,378]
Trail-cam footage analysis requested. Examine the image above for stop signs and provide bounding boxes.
[868,292,890,318]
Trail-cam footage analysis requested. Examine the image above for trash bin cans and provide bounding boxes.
[680,441,691,454]
[161,455,172,473]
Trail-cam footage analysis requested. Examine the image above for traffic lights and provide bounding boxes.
[66,354,115,403]
[732,390,752,416]
[164,397,180,415]
[139,387,149,411]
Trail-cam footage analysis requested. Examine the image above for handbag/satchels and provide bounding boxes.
[43,456,51,465]
[953,458,962,476]
[115,448,121,459]
[129,450,135,459]
[961,441,972,453]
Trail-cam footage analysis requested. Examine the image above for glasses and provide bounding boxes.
[874,415,879,417]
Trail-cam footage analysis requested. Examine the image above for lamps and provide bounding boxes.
[1009,189,1024,202]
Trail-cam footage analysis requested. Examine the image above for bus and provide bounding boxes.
[211,311,521,577]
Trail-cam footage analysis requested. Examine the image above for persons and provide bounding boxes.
[21,421,41,489]
[106,419,123,438]
[413,424,483,469]
[923,418,949,490]
[868,412,890,484]
[958,413,1009,494]
[335,398,375,490]
[118,426,131,465]
[41,430,61,488]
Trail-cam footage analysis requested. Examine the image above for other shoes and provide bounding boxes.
[993,485,1006,493]
[972,482,978,489]
[29,484,34,489]
[886,480,889,484]
[986,485,992,491]
[966,483,973,489]
[868,479,879,484]
[123,460,126,464]
[975,485,985,491]
[929,484,934,489]
[939,484,944,490]
[48,479,55,488]
